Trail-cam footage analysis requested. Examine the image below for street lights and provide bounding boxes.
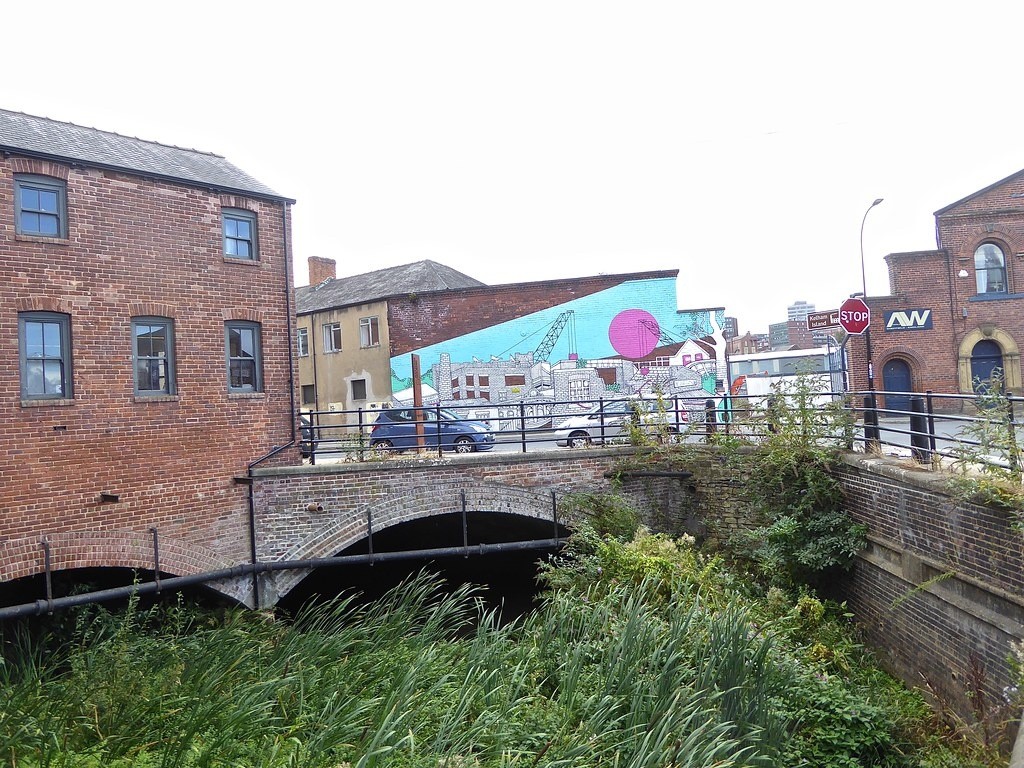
[860,199,884,450]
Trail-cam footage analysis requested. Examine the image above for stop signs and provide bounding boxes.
[838,298,870,335]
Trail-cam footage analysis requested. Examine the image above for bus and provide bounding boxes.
[727,347,851,425]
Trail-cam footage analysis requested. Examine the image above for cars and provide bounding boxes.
[555,394,690,449]
[300,415,319,457]
[369,405,496,458]
